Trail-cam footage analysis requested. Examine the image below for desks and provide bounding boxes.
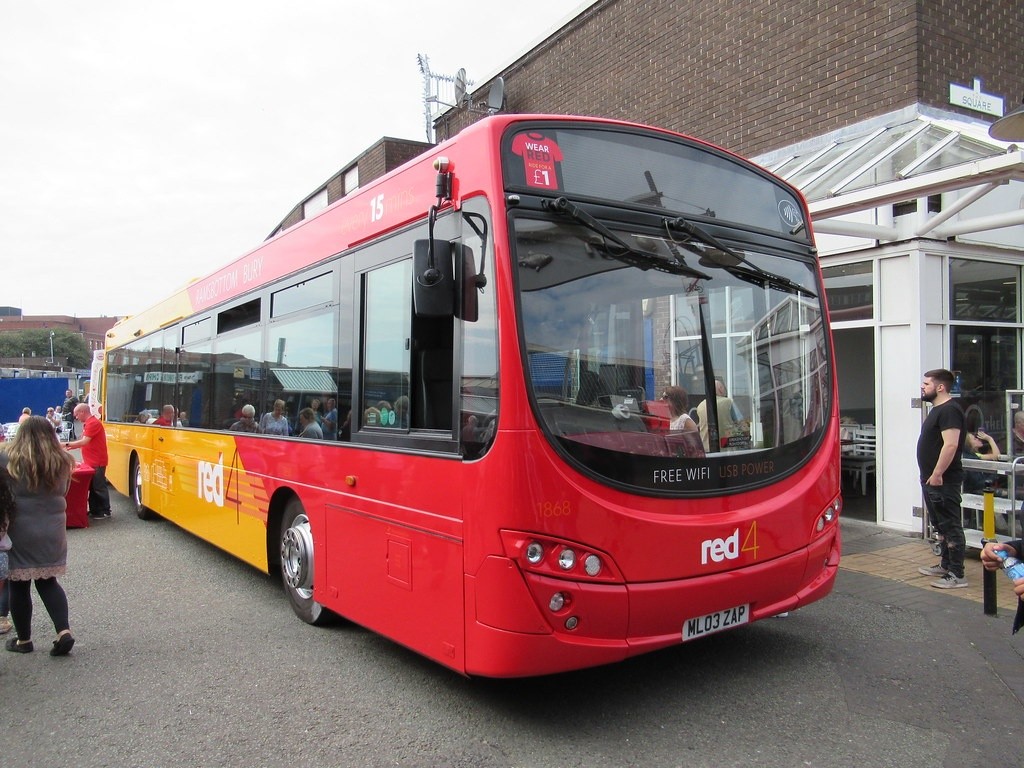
[65,461,95,528]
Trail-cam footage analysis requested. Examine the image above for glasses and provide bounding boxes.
[662,395,671,400]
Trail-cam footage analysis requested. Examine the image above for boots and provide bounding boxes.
[0,616,12,633]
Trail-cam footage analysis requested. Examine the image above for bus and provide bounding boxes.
[89,349,163,428]
[102,113,843,682]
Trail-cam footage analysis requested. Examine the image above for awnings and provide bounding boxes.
[273,369,339,394]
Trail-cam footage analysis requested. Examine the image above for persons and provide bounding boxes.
[996,411,1024,488]
[916,369,968,588]
[230,395,409,440]
[963,430,1006,530]
[0,416,75,656]
[697,379,741,453]
[133,405,189,427]
[980,501,1024,634]
[659,385,698,431]
[60,403,111,519]
[62,389,79,440]
[46,406,63,433]
[462,415,478,441]
[19,407,32,424]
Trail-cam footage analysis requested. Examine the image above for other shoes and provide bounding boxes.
[88,511,111,519]
[50,633,75,656]
[6,637,33,652]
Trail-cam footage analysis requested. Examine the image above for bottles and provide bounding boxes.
[995,549,1024,583]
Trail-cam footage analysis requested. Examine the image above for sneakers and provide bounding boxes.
[930,572,968,588]
[918,563,948,577]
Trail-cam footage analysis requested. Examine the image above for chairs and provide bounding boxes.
[839,423,875,495]
[2,420,74,443]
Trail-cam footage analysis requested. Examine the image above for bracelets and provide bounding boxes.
[66,442,69,446]
